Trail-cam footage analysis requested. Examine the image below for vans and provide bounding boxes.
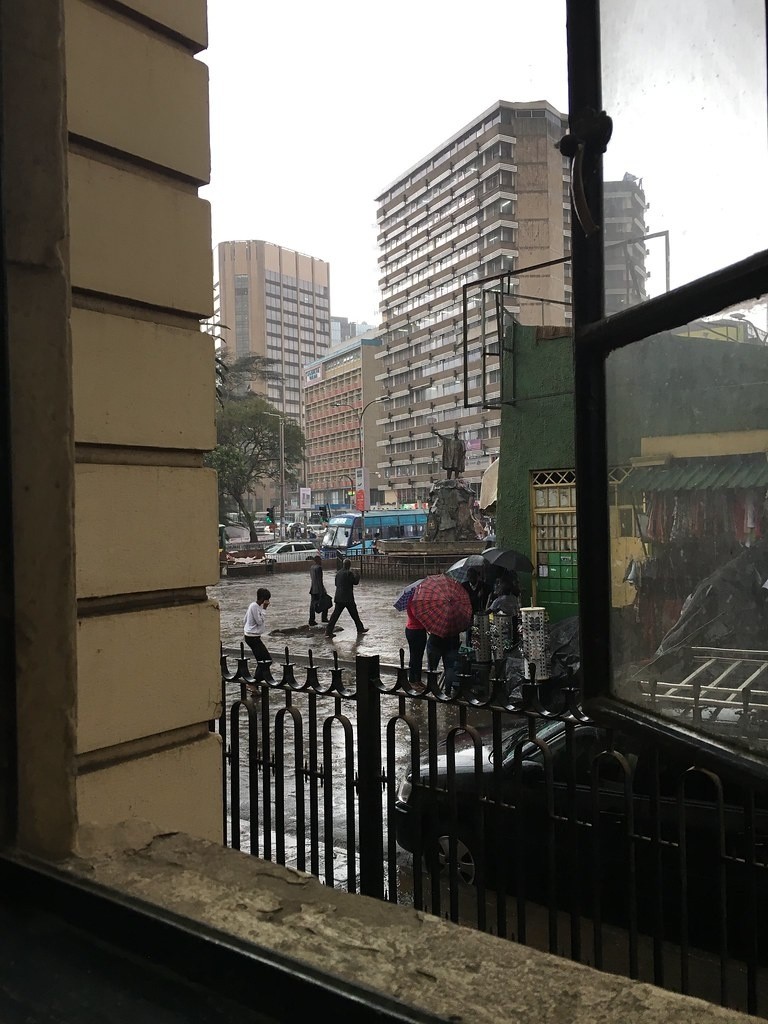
[266,541,321,562]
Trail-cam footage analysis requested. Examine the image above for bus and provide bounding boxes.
[320,508,429,559]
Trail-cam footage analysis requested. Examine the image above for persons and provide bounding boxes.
[323,525,328,534]
[243,589,273,678]
[307,530,316,539]
[405,595,461,694]
[461,569,522,617]
[308,555,369,637]
[435,430,466,479]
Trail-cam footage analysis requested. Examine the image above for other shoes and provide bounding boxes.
[245,683,262,697]
[411,681,426,691]
[318,617,330,622]
[309,620,318,626]
[356,627,369,634]
[323,631,336,638]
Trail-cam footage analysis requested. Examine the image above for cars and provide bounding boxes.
[388,706,768,971]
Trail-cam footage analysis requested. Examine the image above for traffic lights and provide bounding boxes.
[319,506,327,520]
[267,507,272,522]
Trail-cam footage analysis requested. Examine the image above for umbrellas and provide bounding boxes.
[393,546,535,637]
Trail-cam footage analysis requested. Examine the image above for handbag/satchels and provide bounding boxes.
[314,595,334,614]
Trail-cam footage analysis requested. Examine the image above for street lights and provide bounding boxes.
[329,395,394,469]
